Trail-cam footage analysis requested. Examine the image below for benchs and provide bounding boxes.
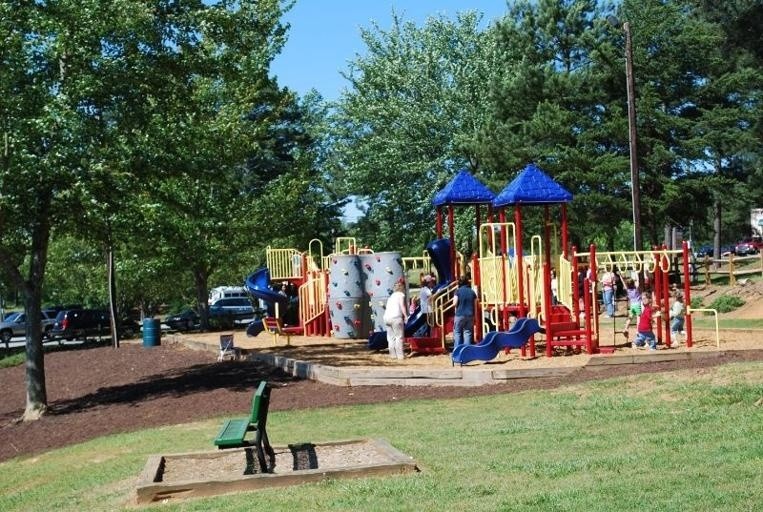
[263,317,301,347]
[214,381,273,468]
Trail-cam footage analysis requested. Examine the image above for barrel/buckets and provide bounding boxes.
[220,334,234,351]
[142,317,160,347]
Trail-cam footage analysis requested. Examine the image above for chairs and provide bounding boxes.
[218,333,243,361]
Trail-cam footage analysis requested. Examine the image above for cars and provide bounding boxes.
[163,302,235,330]
[207,296,267,322]
[691,234,762,258]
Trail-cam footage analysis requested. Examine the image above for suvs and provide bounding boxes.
[51,310,141,341]
[0,308,59,345]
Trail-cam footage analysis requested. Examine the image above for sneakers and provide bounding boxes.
[681,333,688,343]
[671,342,678,348]
[651,348,657,352]
[624,330,628,338]
[636,345,646,350]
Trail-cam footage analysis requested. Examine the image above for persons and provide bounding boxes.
[276,284,290,328]
[450,278,477,351]
[411,275,436,338]
[618,269,688,351]
[406,293,421,316]
[381,281,408,362]
[289,251,302,278]
[551,265,618,320]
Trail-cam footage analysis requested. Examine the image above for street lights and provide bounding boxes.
[606,10,649,293]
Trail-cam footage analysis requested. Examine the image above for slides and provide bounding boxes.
[368,282,451,350]
[451,317,539,363]
[246,269,293,336]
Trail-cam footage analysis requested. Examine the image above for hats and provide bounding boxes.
[423,275,436,282]
[399,279,406,285]
